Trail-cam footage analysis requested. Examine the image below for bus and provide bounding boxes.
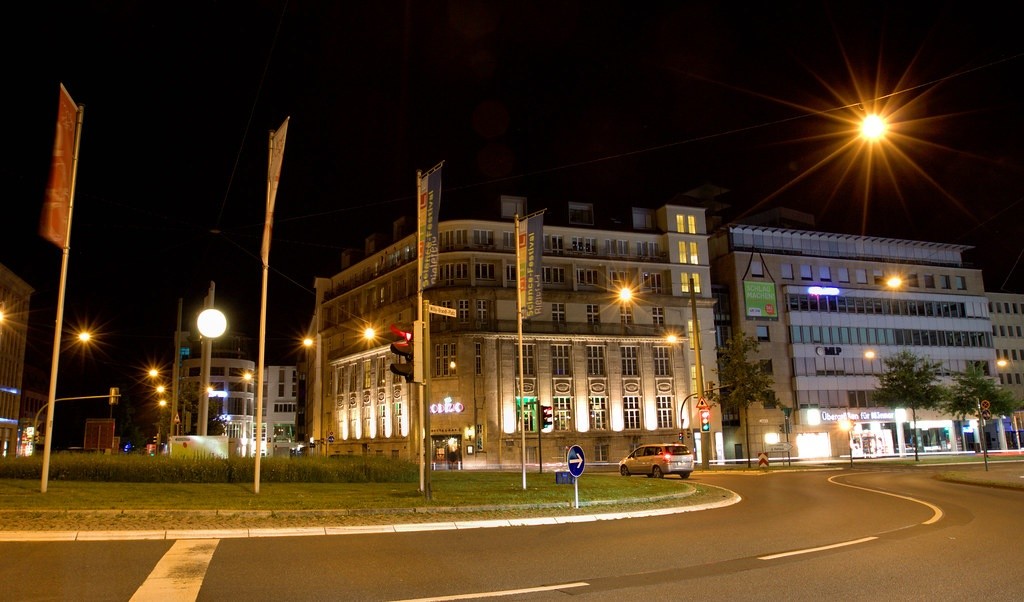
[226,423,301,453]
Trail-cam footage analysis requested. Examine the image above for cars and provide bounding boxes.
[620,443,695,479]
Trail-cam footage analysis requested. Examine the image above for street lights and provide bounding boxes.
[840,421,853,468]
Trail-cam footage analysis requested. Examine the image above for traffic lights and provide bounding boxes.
[784,408,792,417]
[389,320,423,383]
[679,433,683,440]
[687,429,691,439]
[700,410,710,433]
[153,433,160,444]
[541,405,552,429]
[779,424,784,433]
